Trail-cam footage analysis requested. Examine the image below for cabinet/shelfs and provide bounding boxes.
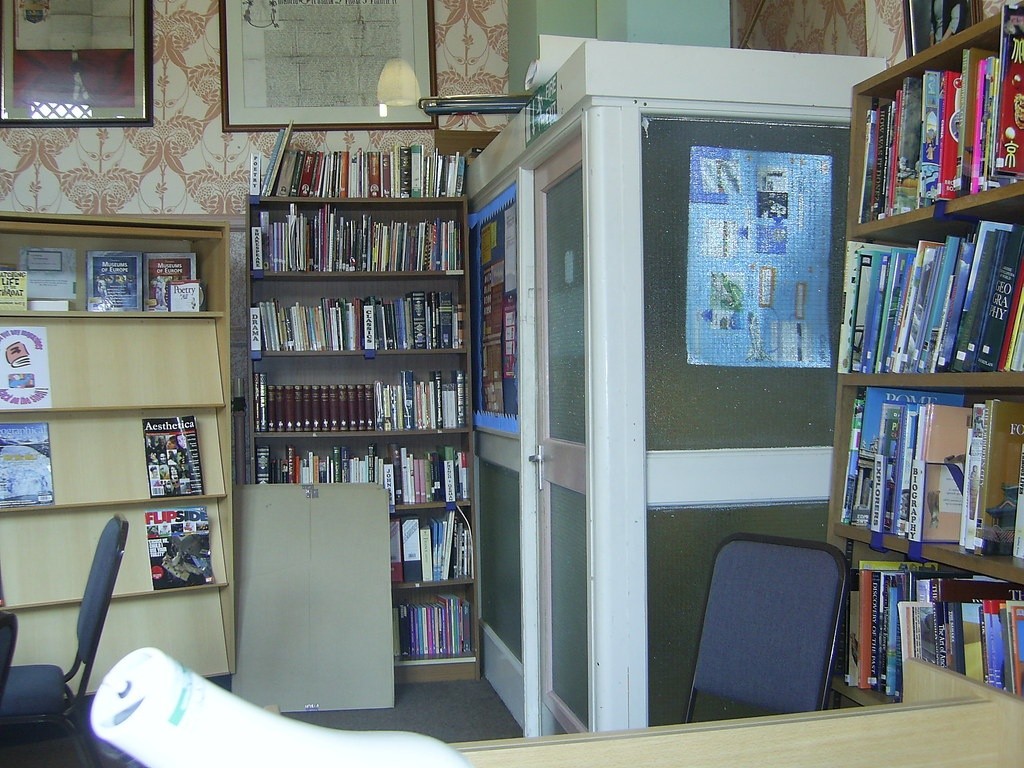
[0,209,233,699]
[823,9,1023,713]
[241,191,483,688]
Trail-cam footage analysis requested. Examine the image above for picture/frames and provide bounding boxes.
[0,0,155,129]
[218,0,441,134]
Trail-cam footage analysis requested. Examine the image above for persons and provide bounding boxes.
[971,465,978,478]
[147,434,190,494]
[928,0,966,47]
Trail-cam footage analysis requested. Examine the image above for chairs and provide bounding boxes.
[681,532,854,724]
[0,512,129,768]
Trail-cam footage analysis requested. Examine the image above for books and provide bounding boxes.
[251,119,471,656]
[0,246,77,310]
[145,505,215,590]
[87,250,142,312]
[0,327,53,410]
[0,423,55,508]
[144,252,199,311]
[841,4,1024,702]
[142,415,204,499]
